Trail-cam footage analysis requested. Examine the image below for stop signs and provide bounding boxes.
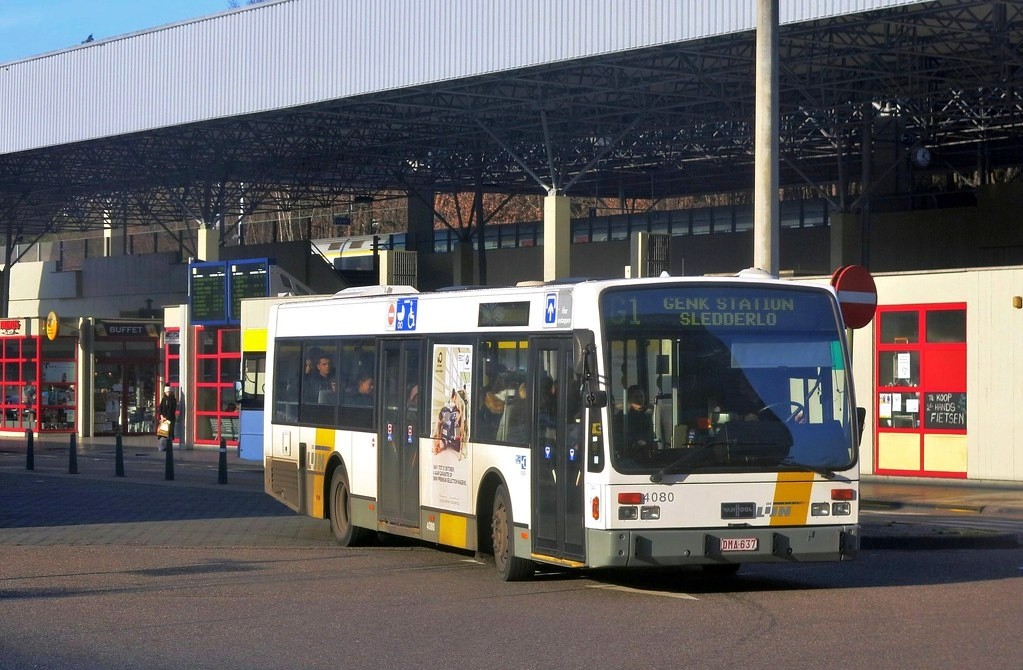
[835,265,878,329]
[384,298,397,331]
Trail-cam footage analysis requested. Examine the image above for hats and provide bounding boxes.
[164,386,171,392]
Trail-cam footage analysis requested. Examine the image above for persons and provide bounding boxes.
[105,388,119,436]
[157,383,176,452]
[222,403,236,419]
[481,350,616,510]
[613,385,653,458]
[707,340,806,434]
[278,348,417,431]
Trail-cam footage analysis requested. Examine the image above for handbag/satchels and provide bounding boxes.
[157,420,171,438]
[158,437,167,451]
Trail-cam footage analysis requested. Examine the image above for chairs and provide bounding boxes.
[286,377,672,447]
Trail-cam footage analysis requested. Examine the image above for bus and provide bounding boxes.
[263,267,866,582]
[233,327,528,461]
